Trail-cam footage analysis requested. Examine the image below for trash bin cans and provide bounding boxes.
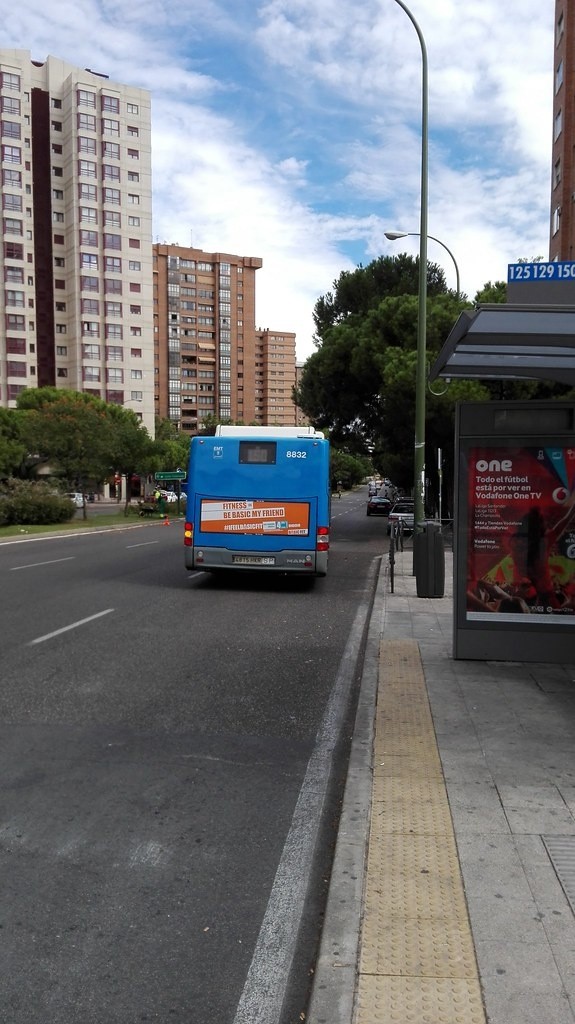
[412,521,445,598]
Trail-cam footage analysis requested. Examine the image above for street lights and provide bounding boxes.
[383,229,460,303]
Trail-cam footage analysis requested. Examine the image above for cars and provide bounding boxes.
[385,503,426,537]
[364,474,394,497]
[391,497,415,513]
[61,492,88,509]
[165,491,187,503]
[366,496,393,517]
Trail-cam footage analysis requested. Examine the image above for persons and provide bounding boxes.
[154,484,166,518]
[338,490,341,498]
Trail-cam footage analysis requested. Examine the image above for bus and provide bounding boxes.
[183,424,332,581]
